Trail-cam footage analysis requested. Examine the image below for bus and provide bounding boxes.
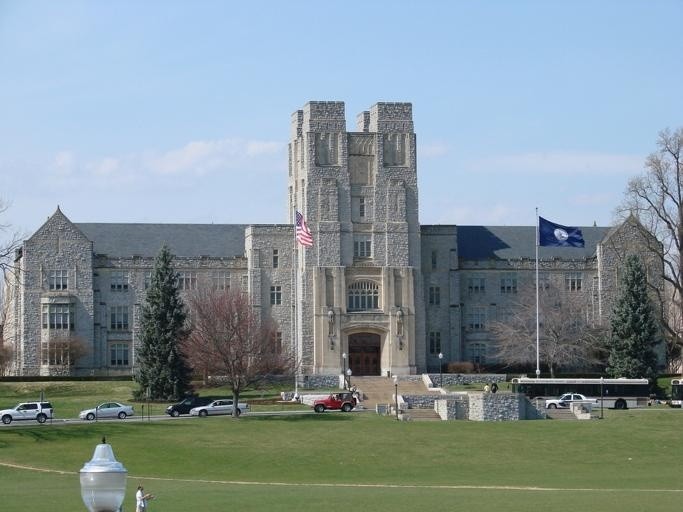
[669,378,683,408]
[506,377,650,410]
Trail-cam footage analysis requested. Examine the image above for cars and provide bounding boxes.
[78,401,134,420]
[544,392,598,409]
[189,398,248,417]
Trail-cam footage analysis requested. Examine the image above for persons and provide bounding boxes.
[333,395,339,400]
[135,485,155,512]
[355,385,363,400]
[491,380,498,393]
[484,384,490,393]
[352,384,359,399]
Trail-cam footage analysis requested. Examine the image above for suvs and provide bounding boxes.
[0,401,54,423]
[311,391,356,413]
[165,397,214,417]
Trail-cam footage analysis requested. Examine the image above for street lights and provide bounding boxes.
[342,352,347,389]
[535,368,540,378]
[438,352,443,387]
[599,377,603,418]
[393,376,399,420]
[77,436,129,512]
[346,368,351,391]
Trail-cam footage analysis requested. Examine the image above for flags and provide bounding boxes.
[295,211,314,248]
[538,216,585,249]
